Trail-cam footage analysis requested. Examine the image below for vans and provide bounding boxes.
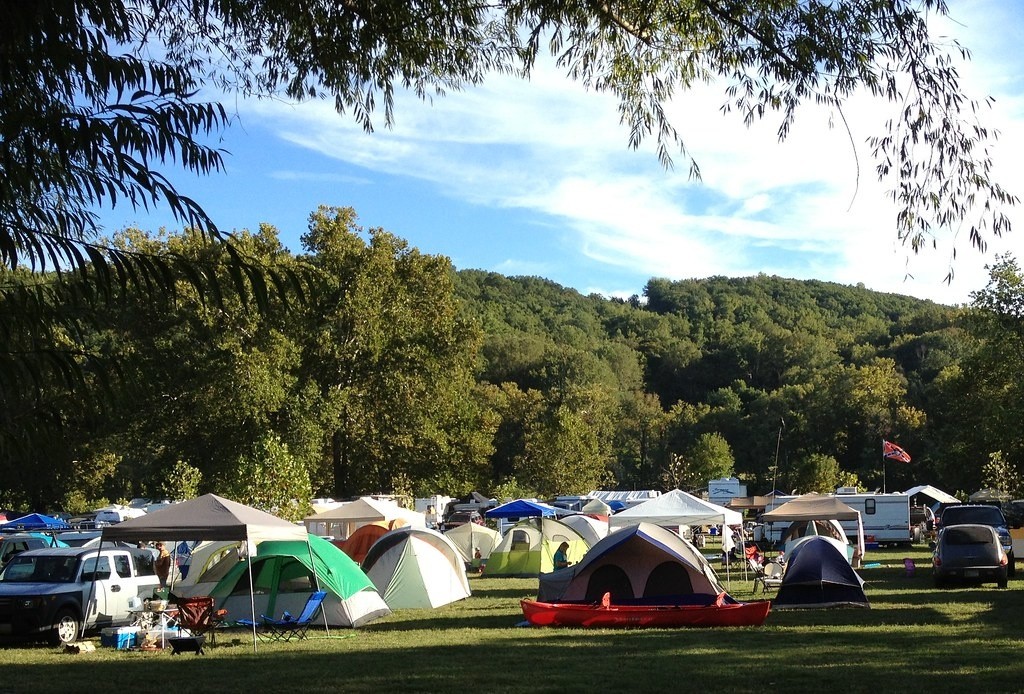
[0,546,166,647]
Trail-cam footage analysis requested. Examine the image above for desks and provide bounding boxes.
[125,609,181,650]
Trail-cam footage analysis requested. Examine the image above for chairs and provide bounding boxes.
[234,611,297,640]
[742,542,769,593]
[763,561,784,592]
[176,596,228,651]
[261,591,328,642]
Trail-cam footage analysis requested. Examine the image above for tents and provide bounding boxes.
[0,480,1013,650]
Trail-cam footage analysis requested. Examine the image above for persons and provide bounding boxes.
[923,505,937,539]
[422,505,439,528]
[137,544,145,550]
[151,543,171,588]
[553,541,573,570]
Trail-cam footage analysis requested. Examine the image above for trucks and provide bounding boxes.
[747,495,910,551]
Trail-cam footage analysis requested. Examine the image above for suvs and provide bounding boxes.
[939,504,1015,576]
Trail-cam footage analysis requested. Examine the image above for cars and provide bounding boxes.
[911,507,935,534]
[931,524,1007,589]
[441,510,485,530]
[0,532,70,570]
[82,537,182,587]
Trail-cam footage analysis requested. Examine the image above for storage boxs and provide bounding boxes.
[100,626,141,651]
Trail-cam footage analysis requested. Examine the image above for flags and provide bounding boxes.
[883,442,911,463]
